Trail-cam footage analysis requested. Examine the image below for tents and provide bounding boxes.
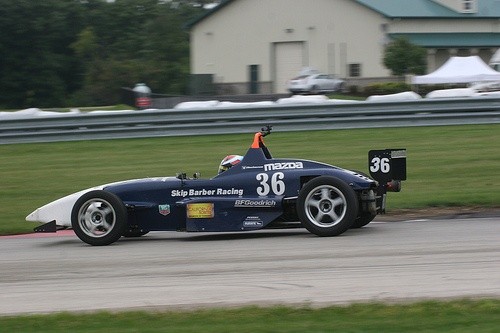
[411,56,500,94]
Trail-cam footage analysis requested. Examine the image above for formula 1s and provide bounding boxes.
[26,125,412,246]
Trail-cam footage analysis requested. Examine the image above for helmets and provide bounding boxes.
[218,155,244,174]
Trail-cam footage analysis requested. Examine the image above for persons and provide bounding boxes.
[219,155,243,175]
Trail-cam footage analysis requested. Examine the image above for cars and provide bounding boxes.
[288,73,344,94]
